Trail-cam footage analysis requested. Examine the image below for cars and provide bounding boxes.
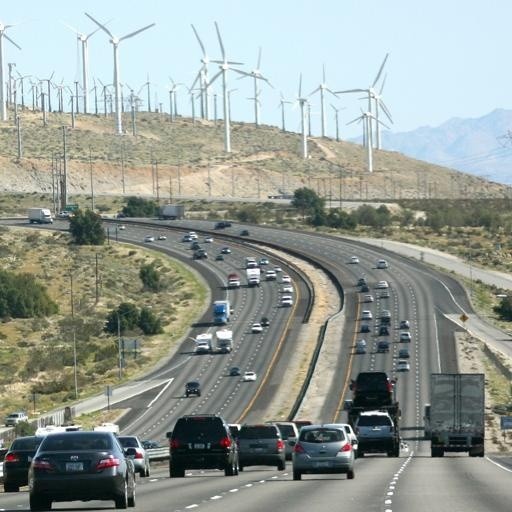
[5,413,28,426]
[59,211,68,217]
[215,221,229,229]
[144,235,166,242]
[183,231,229,261]
[260,258,295,304]
[251,318,269,333]
[3,430,150,511]
[350,256,359,264]
[230,367,256,381]
[377,260,388,269]
[229,420,359,479]
[350,372,393,407]
[355,278,412,371]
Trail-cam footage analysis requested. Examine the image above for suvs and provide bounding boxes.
[167,413,240,477]
[185,382,200,397]
[353,411,396,456]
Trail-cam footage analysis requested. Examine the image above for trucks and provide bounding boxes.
[214,301,229,325]
[196,333,212,353]
[28,208,53,224]
[424,373,485,457]
[216,331,233,353]
[227,257,260,288]
[159,205,183,222]
